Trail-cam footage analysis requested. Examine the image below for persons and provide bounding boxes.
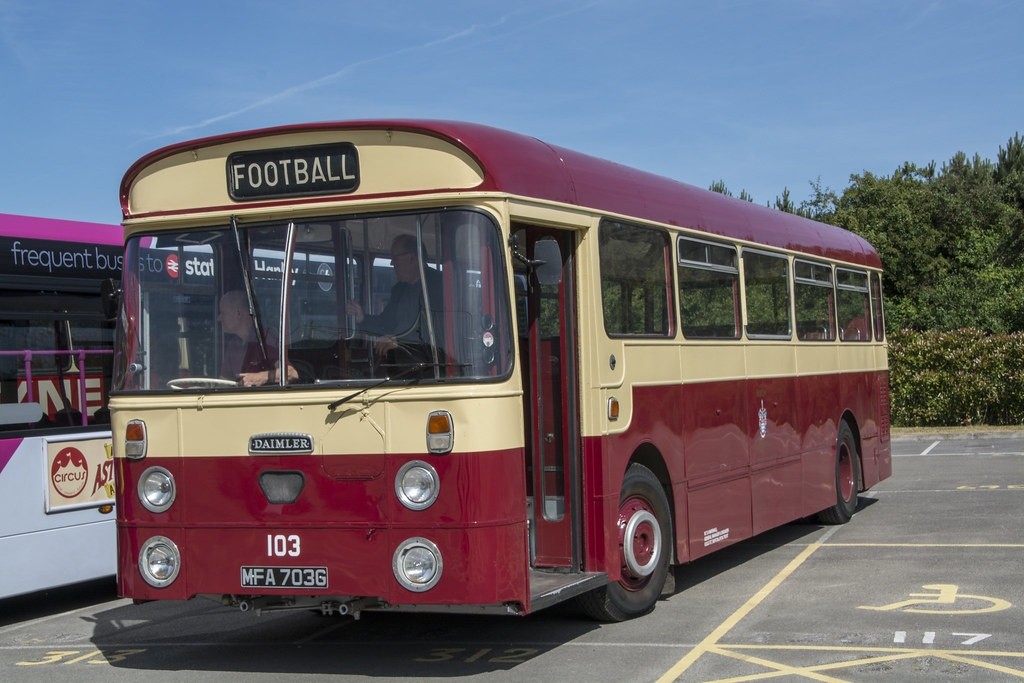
[347,233,446,379]
[216,289,316,388]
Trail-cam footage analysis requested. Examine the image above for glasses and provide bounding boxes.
[390,251,411,259]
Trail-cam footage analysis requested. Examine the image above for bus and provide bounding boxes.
[0,211,530,600]
[98,118,893,624]
